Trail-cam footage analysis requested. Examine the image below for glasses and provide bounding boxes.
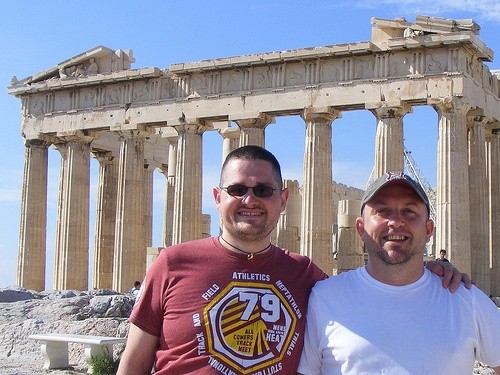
[219,184,283,198]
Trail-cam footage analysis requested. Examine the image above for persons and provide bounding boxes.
[435,249,449,263]
[130,280,141,295]
[297,171,500,375]
[114,144,477,375]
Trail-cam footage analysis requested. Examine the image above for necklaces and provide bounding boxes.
[220,234,271,260]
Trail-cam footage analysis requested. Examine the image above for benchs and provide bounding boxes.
[28,334,128,375]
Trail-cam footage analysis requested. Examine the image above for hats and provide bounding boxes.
[360,171,430,219]
